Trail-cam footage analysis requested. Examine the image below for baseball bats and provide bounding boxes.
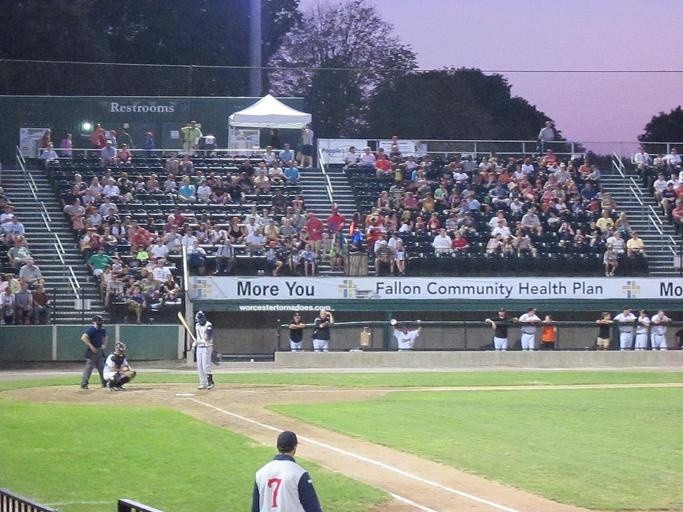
[178,312,196,342]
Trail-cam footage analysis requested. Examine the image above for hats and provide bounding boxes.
[622,305,649,316]
[527,305,537,312]
[497,306,506,313]
[293,313,302,317]
[277,431,299,446]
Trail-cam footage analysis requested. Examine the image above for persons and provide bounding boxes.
[251,430,323,512]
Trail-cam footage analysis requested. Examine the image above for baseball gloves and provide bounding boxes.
[125,371,137,378]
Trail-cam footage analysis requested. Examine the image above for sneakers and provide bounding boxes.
[206,382,214,389]
[198,384,206,389]
[108,386,124,391]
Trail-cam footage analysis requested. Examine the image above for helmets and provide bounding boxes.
[114,343,126,356]
[195,311,208,323]
[92,316,104,324]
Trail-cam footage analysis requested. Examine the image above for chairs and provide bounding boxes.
[94,276,185,324]
[201,244,264,275]
[0,207,50,324]
[635,158,683,234]
[38,152,304,234]
[345,157,395,209]
[403,215,605,272]
